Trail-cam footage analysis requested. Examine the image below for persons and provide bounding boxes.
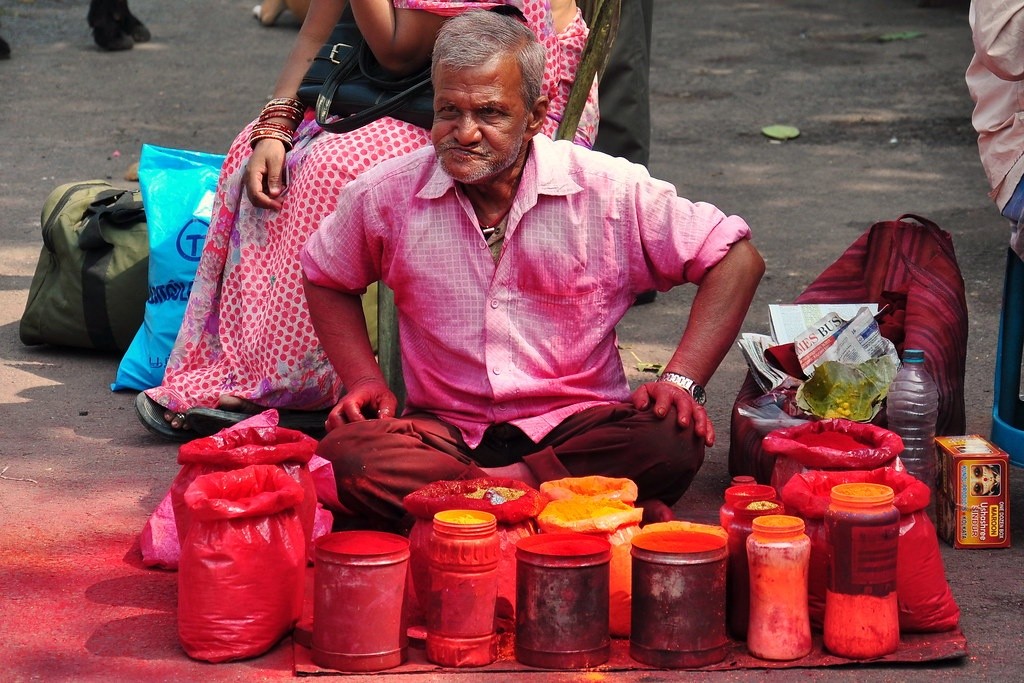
[181,0,600,441]
[576,0,659,308]
[297,6,765,542]
[965,0,1024,230]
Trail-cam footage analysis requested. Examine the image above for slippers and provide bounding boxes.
[135,390,193,443]
[186,407,347,440]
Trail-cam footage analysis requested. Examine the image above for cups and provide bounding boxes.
[629,530,729,667]
[311,531,412,670]
[515,534,613,669]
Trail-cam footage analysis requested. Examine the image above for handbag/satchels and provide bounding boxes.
[111,144,228,392]
[295,1,434,132]
[20,179,151,356]
[728,213,969,486]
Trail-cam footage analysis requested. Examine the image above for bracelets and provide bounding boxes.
[248,98,304,152]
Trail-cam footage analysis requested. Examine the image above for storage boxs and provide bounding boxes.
[935,433,1011,547]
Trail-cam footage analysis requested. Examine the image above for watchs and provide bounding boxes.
[655,372,707,407]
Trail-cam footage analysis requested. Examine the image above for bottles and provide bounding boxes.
[746,513,816,661]
[886,350,940,532]
[426,509,503,667]
[720,476,788,640]
[824,483,900,660]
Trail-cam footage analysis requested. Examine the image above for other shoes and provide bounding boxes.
[86,7,134,51]
[114,10,152,42]
